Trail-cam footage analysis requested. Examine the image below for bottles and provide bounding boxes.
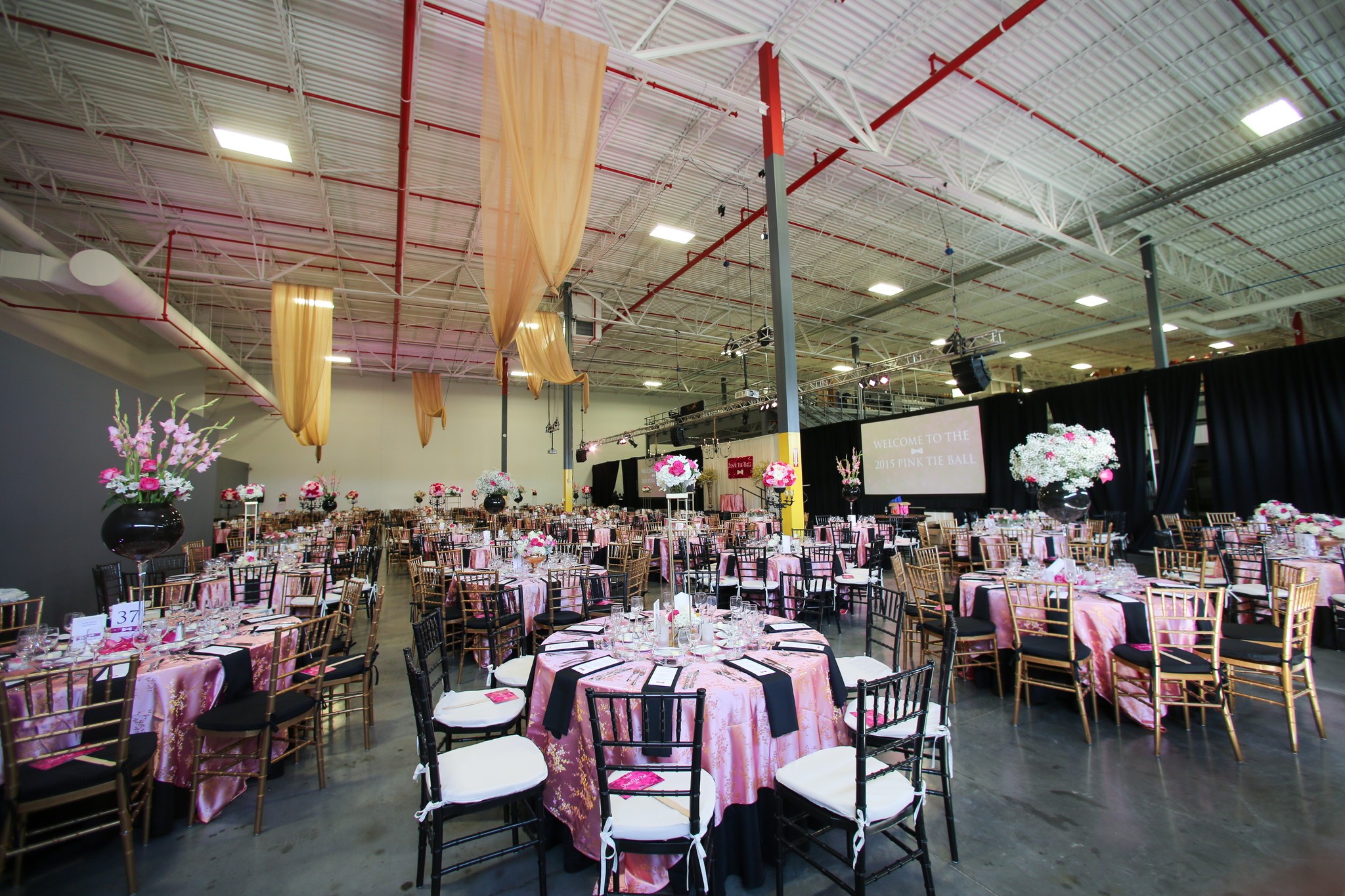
[885,507,888,516]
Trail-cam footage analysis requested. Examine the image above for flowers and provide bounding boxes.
[97,389,239,507]
[1007,419,1122,494]
[279,489,290,498]
[235,482,266,502]
[697,465,719,488]
[687,459,702,487]
[762,461,797,487]
[751,459,769,487]
[835,447,864,486]
[581,485,591,493]
[475,467,520,499]
[641,485,652,492]
[314,470,341,498]
[572,482,580,493]
[654,455,696,493]
[531,488,537,493]
[300,481,324,500]
[220,489,239,505]
[517,486,526,493]
[345,490,358,498]
[429,483,446,497]
[444,486,463,496]
[414,490,425,498]
[472,490,479,496]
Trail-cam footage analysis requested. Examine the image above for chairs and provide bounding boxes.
[0,498,1342,894]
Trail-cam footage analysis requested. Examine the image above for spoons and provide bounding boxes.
[761,642,769,649]
[613,652,634,662]
[560,578,569,581]
[594,638,603,650]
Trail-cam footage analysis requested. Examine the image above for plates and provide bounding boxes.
[690,645,722,655]
[713,623,746,649]
[150,634,218,651]
[98,649,140,660]
[654,647,684,656]
[169,627,196,633]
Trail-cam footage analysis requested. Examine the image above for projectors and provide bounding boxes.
[547,449,557,454]
[735,390,759,402]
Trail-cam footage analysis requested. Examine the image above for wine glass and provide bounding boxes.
[202,519,349,574]
[674,510,705,517]
[635,509,661,514]
[738,513,775,522]
[1232,516,1345,562]
[1004,555,1142,601]
[579,515,620,528]
[662,524,710,536]
[828,515,876,528]
[745,533,816,556]
[972,517,1043,535]
[416,516,578,579]
[604,596,660,661]
[678,621,702,666]
[169,599,197,626]
[727,596,765,657]
[133,618,170,662]
[196,598,243,650]
[506,509,533,515]
[14,612,107,671]
[695,592,717,624]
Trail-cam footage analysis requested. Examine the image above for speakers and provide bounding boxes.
[951,357,990,395]
[575,448,587,463]
[670,426,685,448]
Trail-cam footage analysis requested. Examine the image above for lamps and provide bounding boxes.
[766,490,794,536]
[857,378,867,389]
[879,373,890,385]
[700,417,731,460]
[867,375,879,387]
[644,431,669,467]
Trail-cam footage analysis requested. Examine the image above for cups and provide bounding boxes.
[663,592,674,612]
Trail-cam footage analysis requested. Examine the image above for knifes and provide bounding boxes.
[15,674,73,691]
[224,643,255,646]
[589,667,633,680]
[761,657,794,673]
[715,611,732,617]
[510,580,524,585]
[545,651,594,656]
[688,645,714,651]
[559,631,593,637]
[683,670,699,689]
[765,621,795,624]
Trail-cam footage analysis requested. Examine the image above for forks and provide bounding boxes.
[767,639,775,650]
[614,657,635,662]
[777,648,820,657]
[548,636,585,643]
[530,579,540,583]
[626,666,646,686]
[249,631,275,636]
[169,653,209,661]
[712,666,747,682]
[559,653,593,667]
[63,670,90,684]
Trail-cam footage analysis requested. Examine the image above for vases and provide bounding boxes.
[842,485,860,498]
[1037,484,1089,524]
